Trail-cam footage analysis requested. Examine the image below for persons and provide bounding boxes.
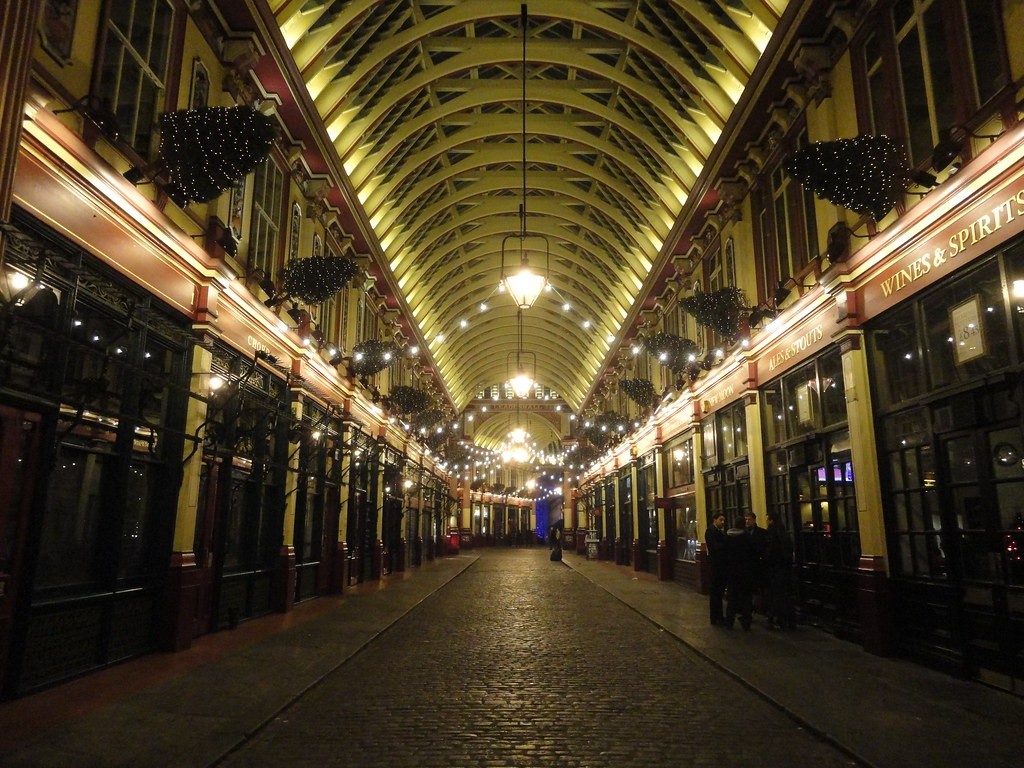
[721,517,754,632]
[743,511,765,548]
[548,525,561,550]
[705,512,729,626]
[760,512,794,631]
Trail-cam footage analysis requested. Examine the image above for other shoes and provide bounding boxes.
[711,616,726,625]
[738,617,751,632]
[726,624,734,630]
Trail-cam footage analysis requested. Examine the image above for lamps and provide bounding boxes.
[508,311,529,442]
[506,204,536,397]
[501,4,550,310]
[606,128,1007,448]
[52,94,429,449]
